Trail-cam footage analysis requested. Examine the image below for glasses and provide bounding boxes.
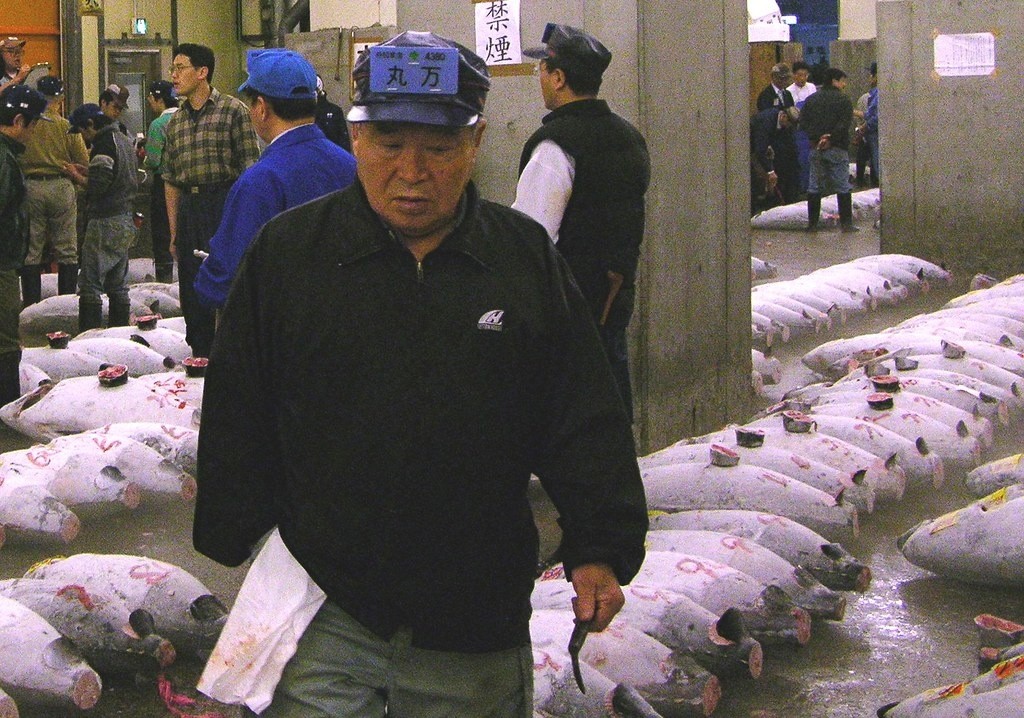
[168,63,195,75]
[4,49,25,56]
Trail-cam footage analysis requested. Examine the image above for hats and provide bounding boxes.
[66,104,103,134]
[863,63,876,75]
[772,61,796,77]
[37,74,64,98]
[238,50,318,99]
[106,82,131,110]
[522,21,612,79]
[346,30,492,126]
[0,36,25,52]
[4,85,53,122]
[148,80,180,99]
[787,107,802,129]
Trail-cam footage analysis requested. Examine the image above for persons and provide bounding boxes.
[0,84,47,406]
[198,49,356,308]
[160,44,261,357]
[0,36,31,91]
[99,83,145,159]
[512,22,651,565]
[194,31,650,718]
[143,81,182,285]
[18,74,89,307]
[750,62,879,232]
[63,102,138,331]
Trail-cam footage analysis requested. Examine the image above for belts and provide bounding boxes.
[26,174,67,182]
[181,182,225,193]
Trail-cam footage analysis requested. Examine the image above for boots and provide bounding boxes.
[0,350,24,428]
[78,302,102,332]
[807,193,822,232]
[836,193,859,233]
[57,262,77,297]
[107,304,130,328]
[20,264,42,307]
